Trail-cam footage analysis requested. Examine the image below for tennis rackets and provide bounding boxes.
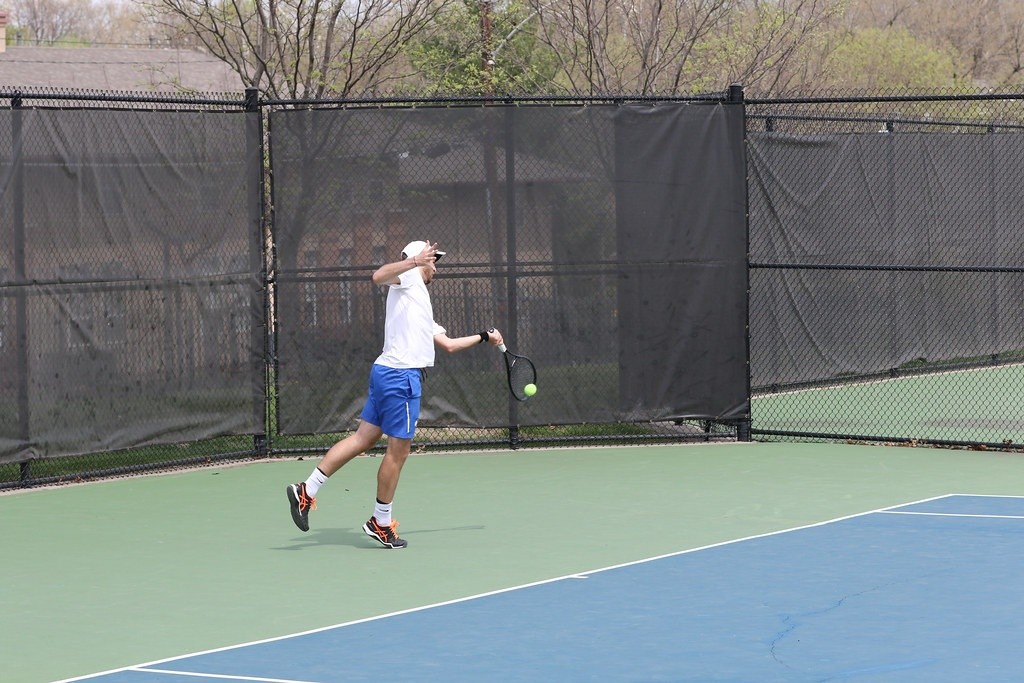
[488,327,537,401]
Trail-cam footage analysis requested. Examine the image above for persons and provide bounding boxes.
[285,240,504,548]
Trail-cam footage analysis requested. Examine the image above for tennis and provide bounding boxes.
[523,383,537,398]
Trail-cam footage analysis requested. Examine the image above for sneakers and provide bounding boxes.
[287,481,316,532]
[362,515,408,548]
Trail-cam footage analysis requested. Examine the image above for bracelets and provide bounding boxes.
[478,331,489,344]
[413,256,417,267]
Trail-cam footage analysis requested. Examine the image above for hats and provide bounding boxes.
[401,240,446,263]
